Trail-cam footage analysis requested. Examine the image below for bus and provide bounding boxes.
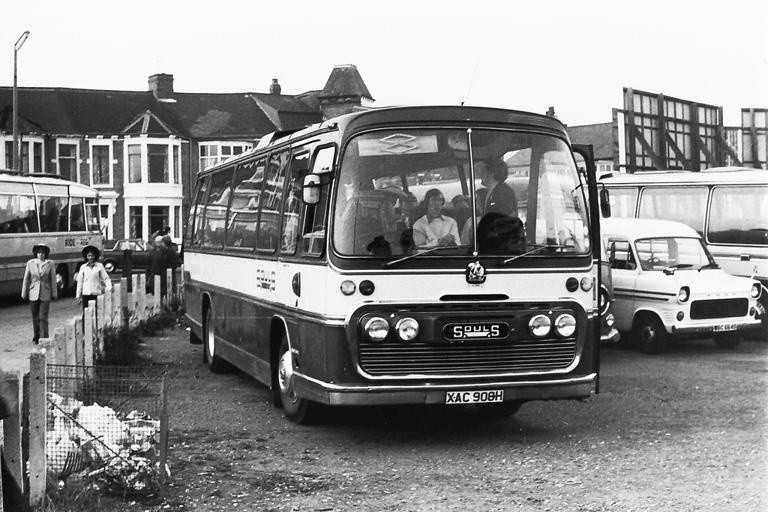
[182,105,622,425]
[566,165,768,343]
[0,170,108,298]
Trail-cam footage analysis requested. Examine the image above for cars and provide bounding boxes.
[103,230,182,273]
[546,216,764,355]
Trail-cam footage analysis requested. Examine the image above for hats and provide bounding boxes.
[82,245,100,261]
[33,243,50,257]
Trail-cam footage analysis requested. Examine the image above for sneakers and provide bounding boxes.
[32,338,39,344]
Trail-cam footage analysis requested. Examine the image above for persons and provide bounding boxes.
[21,244,57,344]
[76,246,112,347]
[413,158,518,254]
[146,226,178,298]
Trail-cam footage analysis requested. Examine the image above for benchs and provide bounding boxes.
[393,205,477,228]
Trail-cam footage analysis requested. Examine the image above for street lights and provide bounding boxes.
[13,30,30,170]
